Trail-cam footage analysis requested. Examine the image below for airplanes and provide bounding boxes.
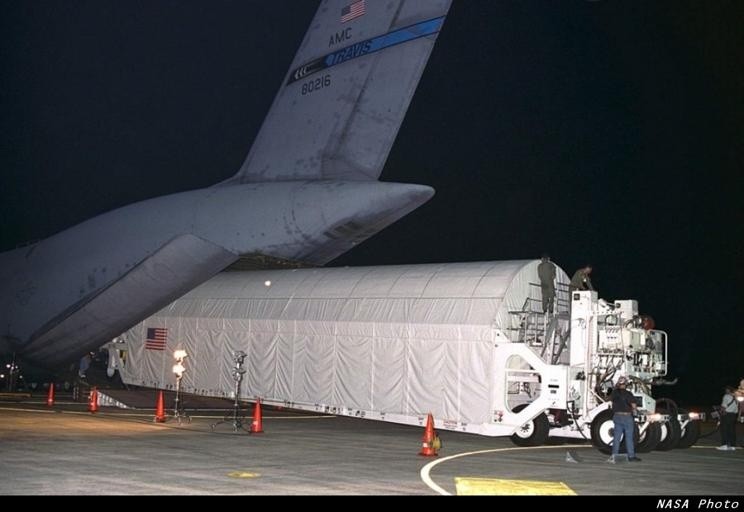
[1,0,453,392]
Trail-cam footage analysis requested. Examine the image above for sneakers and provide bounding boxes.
[629,457,642,462]
[715,445,737,451]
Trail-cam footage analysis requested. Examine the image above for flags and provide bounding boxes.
[145,327,168,351]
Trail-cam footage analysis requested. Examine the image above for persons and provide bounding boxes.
[607,376,641,464]
[713,386,738,451]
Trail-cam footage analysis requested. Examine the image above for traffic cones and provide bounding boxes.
[416,412,439,458]
[87,386,99,412]
[249,397,264,434]
[45,383,55,408]
[152,389,166,423]
